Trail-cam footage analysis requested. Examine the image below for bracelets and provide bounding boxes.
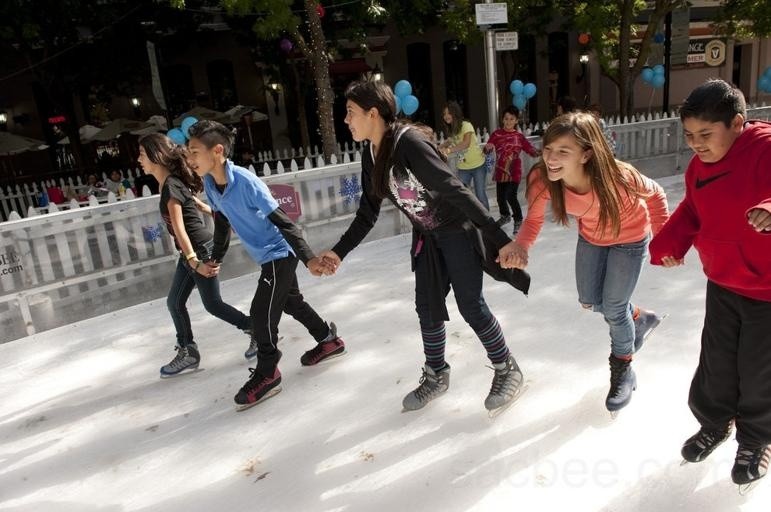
[186,252,204,273]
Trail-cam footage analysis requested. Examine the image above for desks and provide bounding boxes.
[36,196,126,211]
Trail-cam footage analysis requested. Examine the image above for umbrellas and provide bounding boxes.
[3,92,270,161]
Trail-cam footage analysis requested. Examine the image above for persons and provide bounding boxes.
[136,134,283,376]
[321,79,531,422]
[648,78,771,495]
[81,172,103,194]
[495,109,684,418]
[189,121,347,412]
[103,171,124,191]
[483,106,543,235]
[589,108,617,159]
[436,101,489,211]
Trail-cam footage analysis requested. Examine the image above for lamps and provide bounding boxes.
[372,62,384,82]
[577,49,589,84]
[131,95,143,117]
[269,77,281,116]
[0,111,8,131]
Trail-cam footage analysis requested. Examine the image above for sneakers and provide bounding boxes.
[513,220,522,235]
[495,215,512,226]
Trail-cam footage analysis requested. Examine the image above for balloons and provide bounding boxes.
[377,80,425,116]
[509,79,539,110]
[165,115,202,147]
[642,64,673,86]
[759,69,771,95]
